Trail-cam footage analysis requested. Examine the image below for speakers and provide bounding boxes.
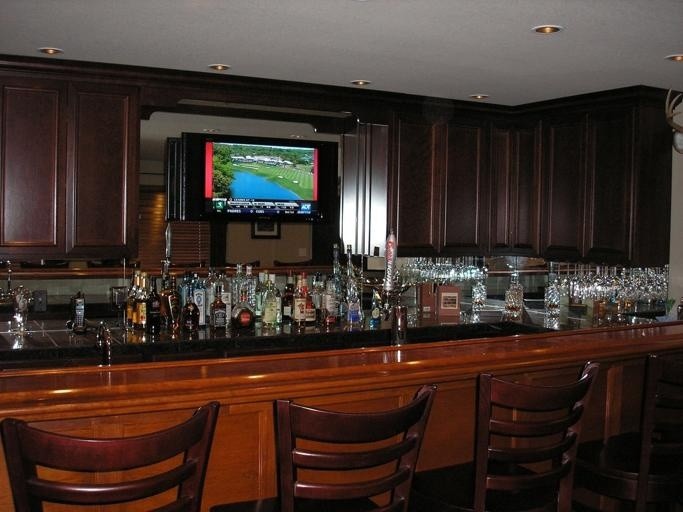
[328,143,338,222]
[167,138,177,220]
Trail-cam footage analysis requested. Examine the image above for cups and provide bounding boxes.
[390,305,407,332]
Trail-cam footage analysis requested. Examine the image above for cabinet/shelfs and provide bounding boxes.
[359,108,436,257]
[438,105,491,256]
[490,103,541,256]
[0,74,141,260]
[543,100,671,269]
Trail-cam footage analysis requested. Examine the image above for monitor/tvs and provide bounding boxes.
[180,132,329,224]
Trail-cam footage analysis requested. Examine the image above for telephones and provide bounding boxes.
[71,292,87,335]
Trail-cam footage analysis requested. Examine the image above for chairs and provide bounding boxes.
[572,353,681,511]
[412,363,599,512]
[0,401,221,512]
[208,385,437,512]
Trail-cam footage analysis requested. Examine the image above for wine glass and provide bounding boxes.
[558,262,670,303]
[404,256,490,283]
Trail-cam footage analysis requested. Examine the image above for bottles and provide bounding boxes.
[543,272,562,330]
[126,270,161,334]
[504,274,524,322]
[160,244,383,335]
[471,280,487,312]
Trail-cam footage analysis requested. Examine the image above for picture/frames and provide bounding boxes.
[437,286,461,316]
[250,222,281,239]
